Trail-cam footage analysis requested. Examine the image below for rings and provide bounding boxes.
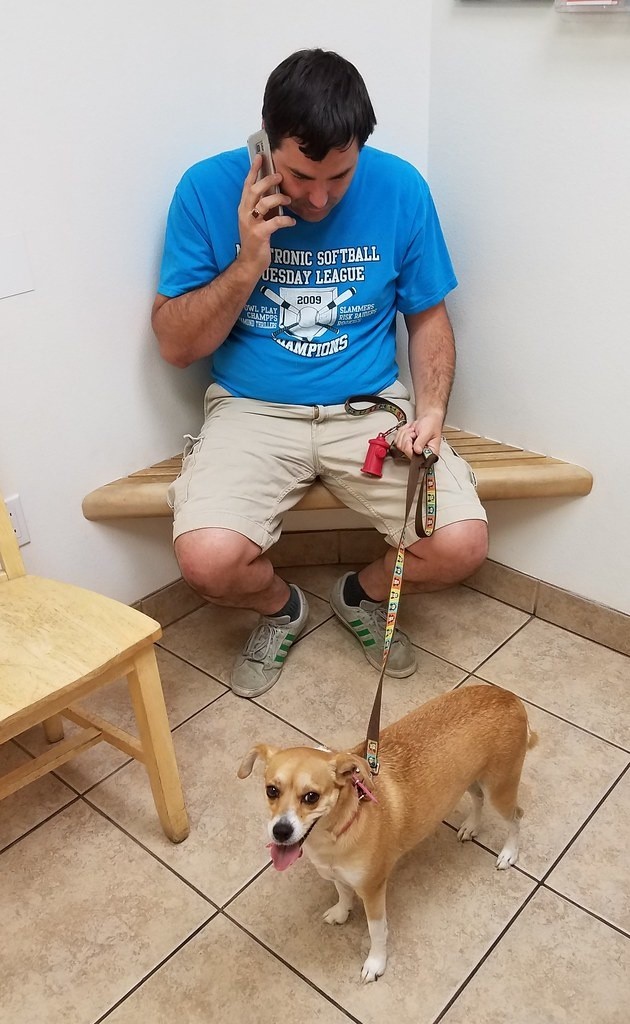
[251,208,265,221]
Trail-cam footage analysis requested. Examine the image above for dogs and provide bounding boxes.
[237,684,540,986]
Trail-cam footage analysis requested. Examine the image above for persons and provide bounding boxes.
[151,49,489,698]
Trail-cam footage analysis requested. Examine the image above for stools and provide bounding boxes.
[83,409,589,517]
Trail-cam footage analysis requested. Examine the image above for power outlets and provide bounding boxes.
[4,495,30,548]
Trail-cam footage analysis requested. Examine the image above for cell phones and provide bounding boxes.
[248,129,283,221]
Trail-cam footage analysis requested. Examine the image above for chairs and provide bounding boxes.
[0,492,190,847]
